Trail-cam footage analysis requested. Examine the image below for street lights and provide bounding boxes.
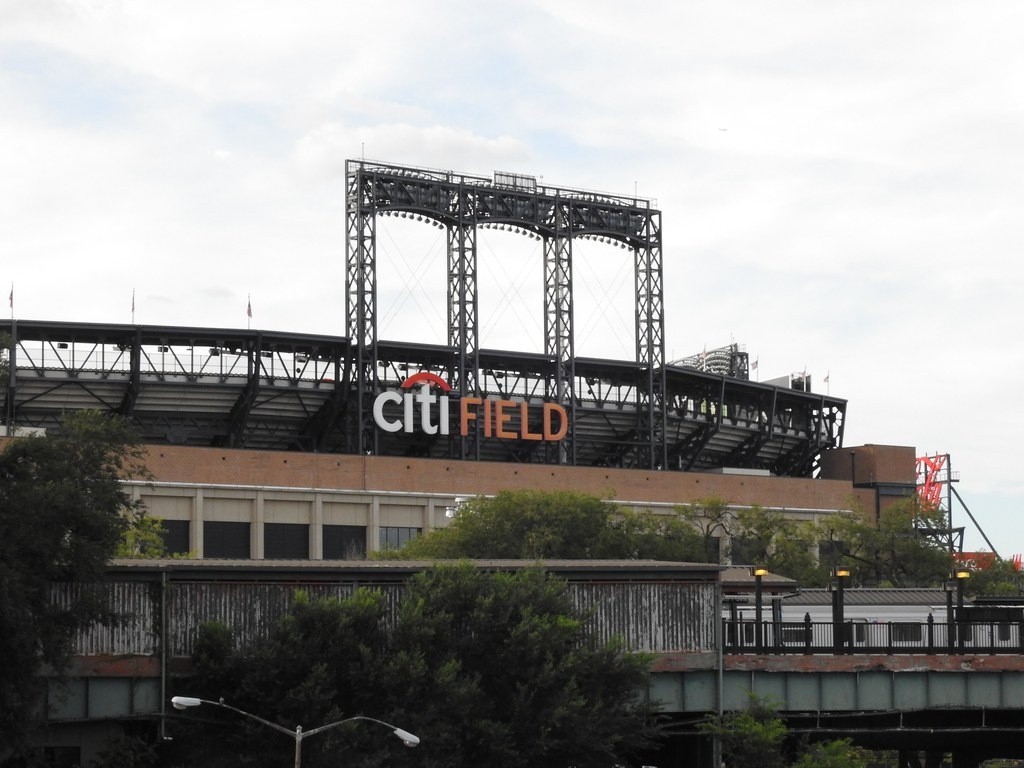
[170,695,420,768]
